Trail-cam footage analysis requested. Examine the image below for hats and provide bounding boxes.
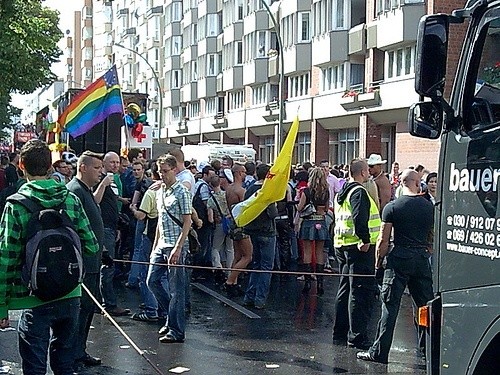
[367,154,387,165]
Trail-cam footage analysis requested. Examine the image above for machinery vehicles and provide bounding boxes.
[34,87,148,160]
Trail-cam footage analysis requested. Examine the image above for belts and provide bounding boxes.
[275,215,288,220]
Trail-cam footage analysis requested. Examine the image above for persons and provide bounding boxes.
[332,158,382,348]
[146,154,193,343]
[65,151,104,365]
[356,169,434,363]
[422,172,438,293]
[0,137,441,323]
[0,139,100,375]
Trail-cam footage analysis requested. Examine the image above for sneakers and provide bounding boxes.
[138,313,159,322]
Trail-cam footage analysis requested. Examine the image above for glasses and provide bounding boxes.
[156,167,175,174]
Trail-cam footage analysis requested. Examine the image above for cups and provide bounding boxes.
[107,173,113,181]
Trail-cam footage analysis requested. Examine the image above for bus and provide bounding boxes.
[406,0,500,375]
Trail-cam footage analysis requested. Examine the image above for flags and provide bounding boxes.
[230,115,299,227]
[57,64,123,139]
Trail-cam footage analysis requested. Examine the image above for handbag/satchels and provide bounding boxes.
[299,201,317,218]
[188,229,202,253]
[223,218,233,234]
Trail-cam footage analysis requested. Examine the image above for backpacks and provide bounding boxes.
[192,183,209,229]
[6,193,82,299]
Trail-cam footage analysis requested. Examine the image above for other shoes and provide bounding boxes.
[75,354,101,366]
[272,263,332,294]
[107,308,132,316]
[159,328,185,344]
[357,351,388,362]
[213,267,248,297]
[348,340,374,350]
[415,349,426,358]
[158,326,169,336]
